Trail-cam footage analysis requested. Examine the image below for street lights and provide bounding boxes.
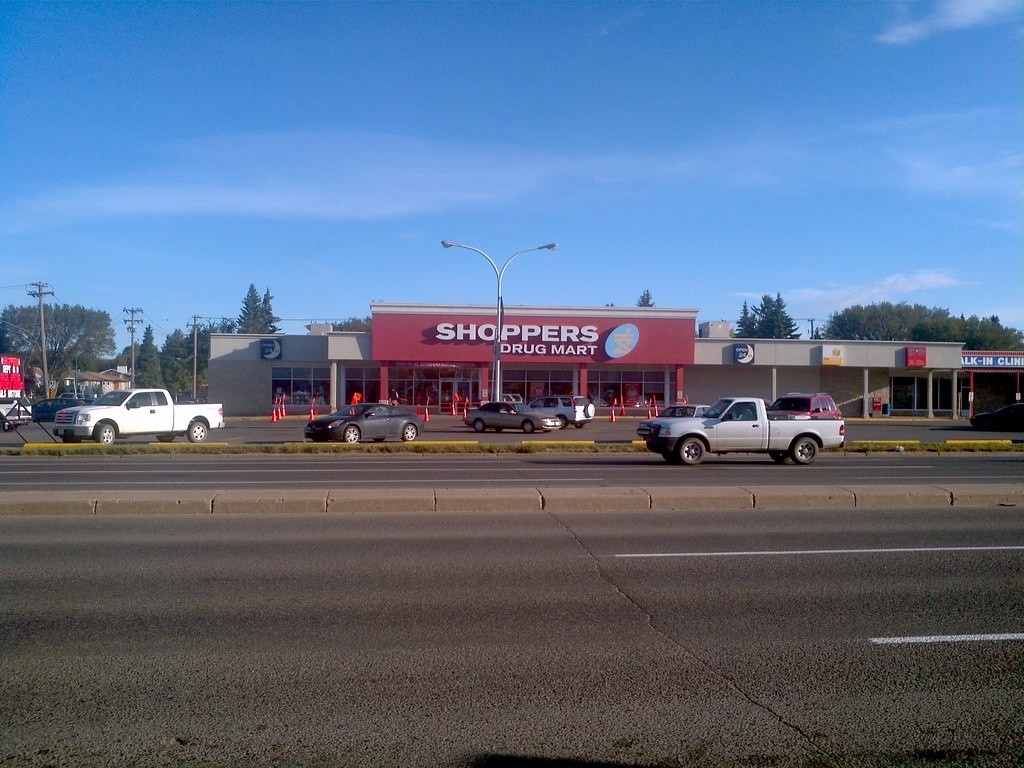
[440,240,559,404]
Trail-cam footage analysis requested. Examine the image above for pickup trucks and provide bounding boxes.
[168,390,208,405]
[52,388,225,446]
[646,397,845,465]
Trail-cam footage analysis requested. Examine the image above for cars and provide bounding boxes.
[305,403,424,443]
[31,398,88,423]
[465,401,564,434]
[635,404,711,443]
[970,403,1024,433]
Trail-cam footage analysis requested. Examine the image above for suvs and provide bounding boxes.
[527,395,595,430]
[767,392,841,421]
[1,395,33,432]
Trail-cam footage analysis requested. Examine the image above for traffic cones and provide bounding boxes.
[450,403,457,416]
[460,407,468,421]
[280,402,286,416]
[309,409,314,421]
[423,407,430,422]
[647,409,652,420]
[619,405,625,416]
[416,407,421,415]
[609,409,616,423]
[271,409,277,422]
[276,407,282,420]
[654,406,659,417]
